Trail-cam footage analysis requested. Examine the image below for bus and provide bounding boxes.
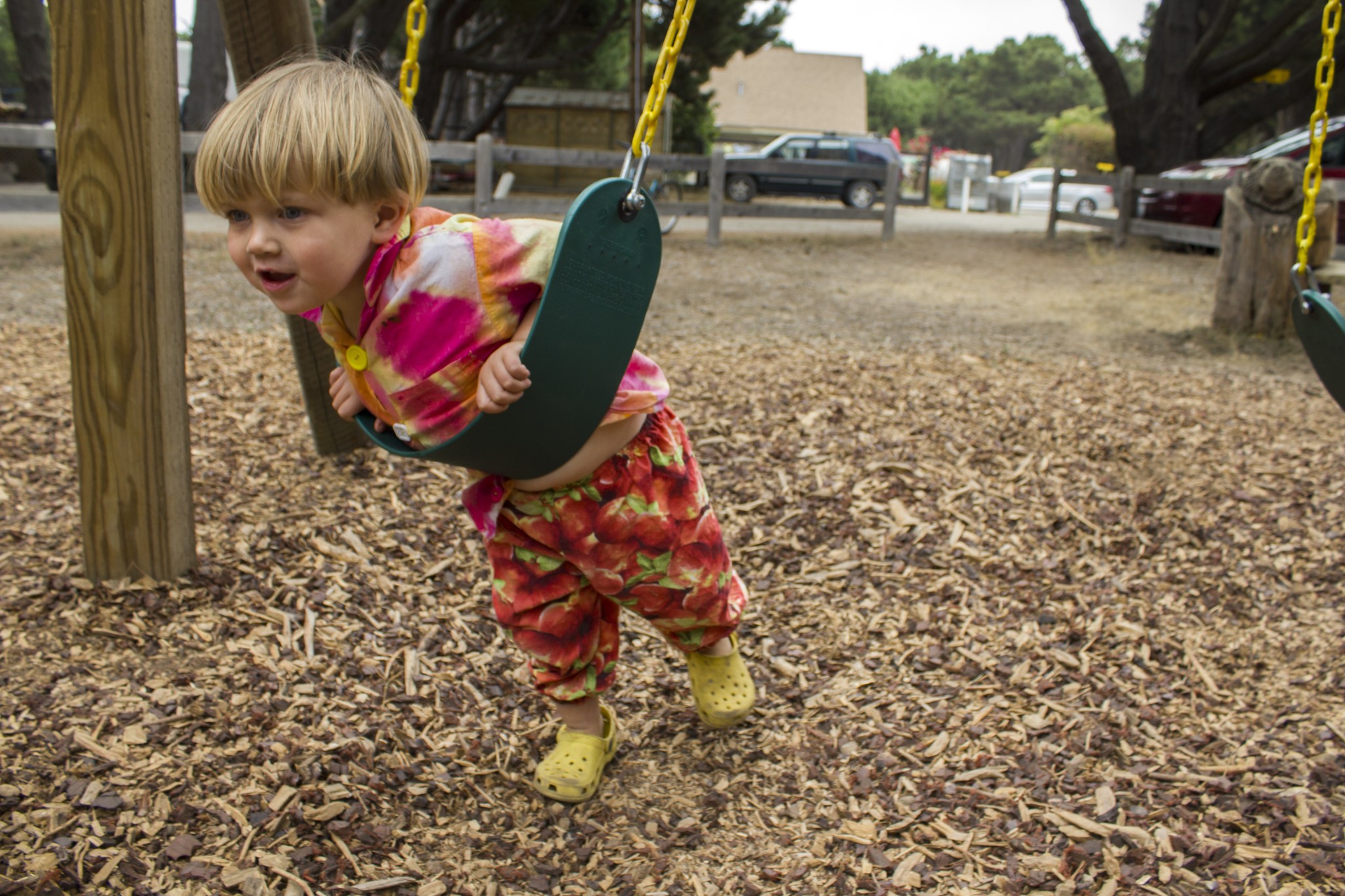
[724,130,904,209]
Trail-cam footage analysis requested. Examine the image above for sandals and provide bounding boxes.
[533,703,618,804]
[685,632,756,729]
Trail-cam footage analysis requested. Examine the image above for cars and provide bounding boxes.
[1000,167,1115,216]
[1137,116,1344,255]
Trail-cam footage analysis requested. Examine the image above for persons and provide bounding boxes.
[194,42,757,806]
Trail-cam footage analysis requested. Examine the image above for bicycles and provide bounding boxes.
[611,139,684,235]
